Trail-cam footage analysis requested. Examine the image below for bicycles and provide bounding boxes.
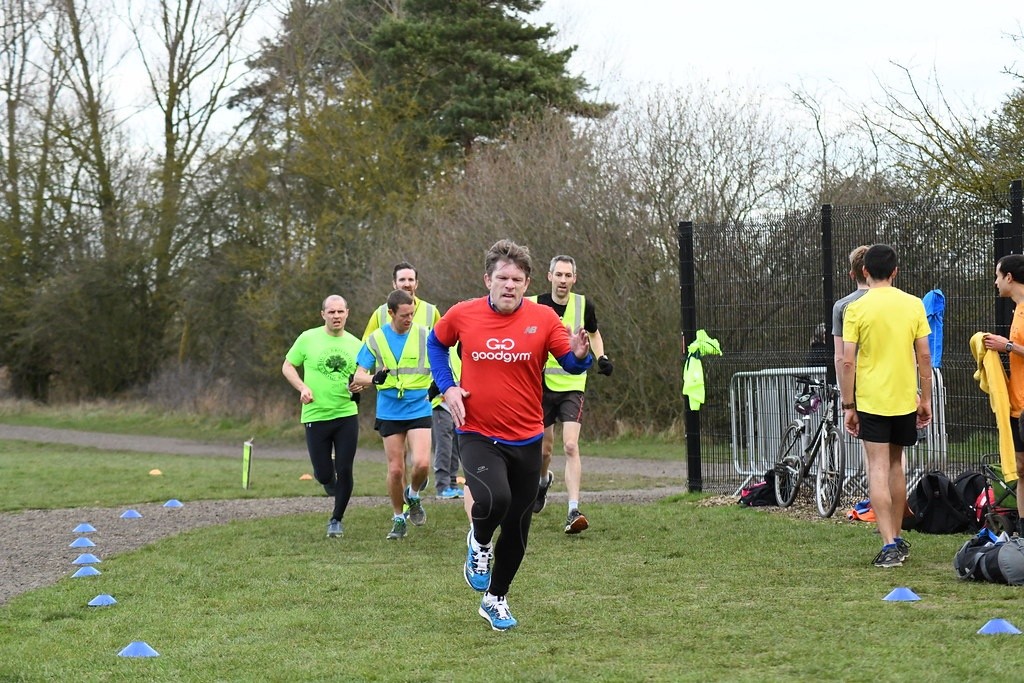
[772,375,846,517]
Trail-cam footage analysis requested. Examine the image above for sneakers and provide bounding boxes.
[464,528,493,592]
[872,545,903,567]
[322,455,337,496]
[565,508,589,534]
[326,518,344,538]
[478,589,517,632]
[435,487,458,499]
[532,469,555,513]
[404,485,426,526]
[384,515,409,539]
[453,488,465,497]
[896,538,911,562]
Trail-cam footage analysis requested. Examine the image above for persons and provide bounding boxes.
[831,245,906,473]
[354,290,441,539]
[282,294,365,538]
[427,239,589,632]
[529,256,612,533]
[983,254,1024,540]
[843,244,933,566]
[431,345,464,499]
[362,262,441,519]
[809,323,826,367]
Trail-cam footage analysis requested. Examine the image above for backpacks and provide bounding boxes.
[741,471,790,506]
[900,470,1009,534]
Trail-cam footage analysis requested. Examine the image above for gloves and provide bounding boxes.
[372,369,391,385]
[597,356,614,377]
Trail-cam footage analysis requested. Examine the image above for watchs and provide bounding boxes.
[598,355,608,363]
[1006,341,1013,352]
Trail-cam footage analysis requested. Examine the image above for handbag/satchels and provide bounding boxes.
[952,526,1024,586]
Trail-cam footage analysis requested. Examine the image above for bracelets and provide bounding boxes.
[842,402,855,410]
